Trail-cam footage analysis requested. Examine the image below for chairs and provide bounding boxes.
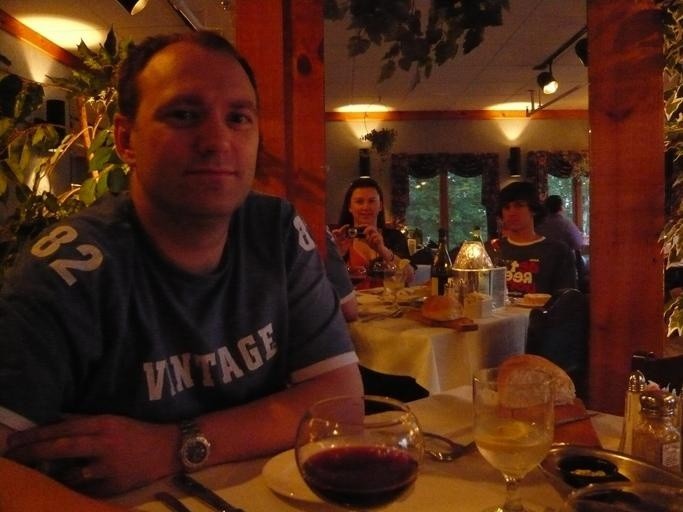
[525,287,587,403]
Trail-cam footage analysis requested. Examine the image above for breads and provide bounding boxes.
[422,296,463,321]
[498,354,576,409]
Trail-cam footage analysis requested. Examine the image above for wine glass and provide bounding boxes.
[470,365,557,512]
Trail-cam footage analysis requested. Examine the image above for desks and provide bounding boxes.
[347,284,548,396]
[117,383,680,510]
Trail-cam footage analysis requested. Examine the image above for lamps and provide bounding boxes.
[530,26,587,94]
[359,148,371,179]
[117,0,148,16]
[507,147,521,178]
[452,225,497,274]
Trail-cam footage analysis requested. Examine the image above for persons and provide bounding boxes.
[483,181,575,355]
[328,176,416,289]
[324,231,360,323]
[535,194,584,293]
[0,29,366,512]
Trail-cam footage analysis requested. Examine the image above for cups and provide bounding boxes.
[346,266,368,286]
[294,393,426,512]
[369,259,397,278]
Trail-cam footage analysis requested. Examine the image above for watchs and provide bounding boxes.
[179,418,211,473]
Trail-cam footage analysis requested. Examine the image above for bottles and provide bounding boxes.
[429,227,453,296]
[619,369,648,458]
[470,226,484,244]
[631,389,683,473]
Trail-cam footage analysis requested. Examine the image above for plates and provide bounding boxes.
[538,443,683,511]
[514,297,545,308]
[261,448,326,504]
[405,392,474,448]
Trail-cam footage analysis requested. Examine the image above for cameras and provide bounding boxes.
[345,226,366,239]
